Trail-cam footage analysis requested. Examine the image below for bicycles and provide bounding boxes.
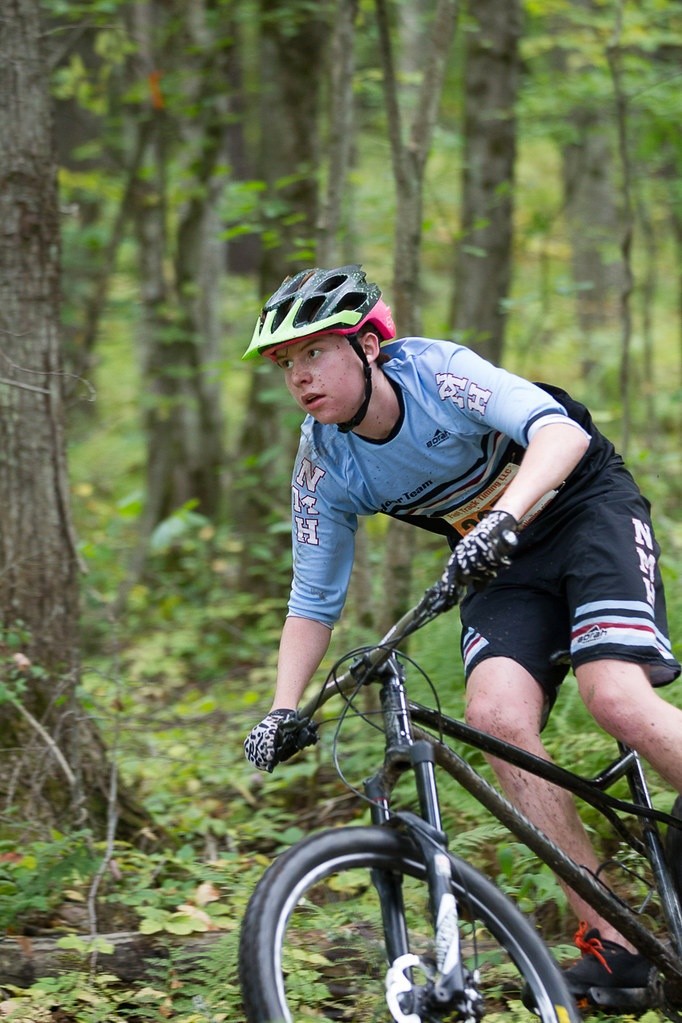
[234,527,682,1023]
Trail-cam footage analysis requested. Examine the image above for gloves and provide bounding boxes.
[439,510,520,600]
[244,710,312,773]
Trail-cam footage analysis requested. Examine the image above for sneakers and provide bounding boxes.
[520,921,653,1017]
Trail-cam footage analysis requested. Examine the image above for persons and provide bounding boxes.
[242,261,681,1013]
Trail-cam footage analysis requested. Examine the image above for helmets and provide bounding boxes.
[242,264,396,368]
